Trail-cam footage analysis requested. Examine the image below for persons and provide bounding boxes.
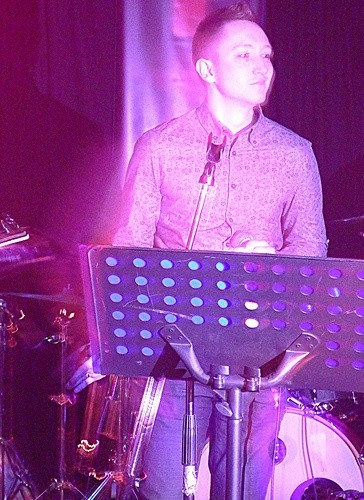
[106,0,331,499]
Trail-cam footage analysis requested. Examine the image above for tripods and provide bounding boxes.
[0,299,146,500]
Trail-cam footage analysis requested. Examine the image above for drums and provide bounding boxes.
[192,403,363,500]
